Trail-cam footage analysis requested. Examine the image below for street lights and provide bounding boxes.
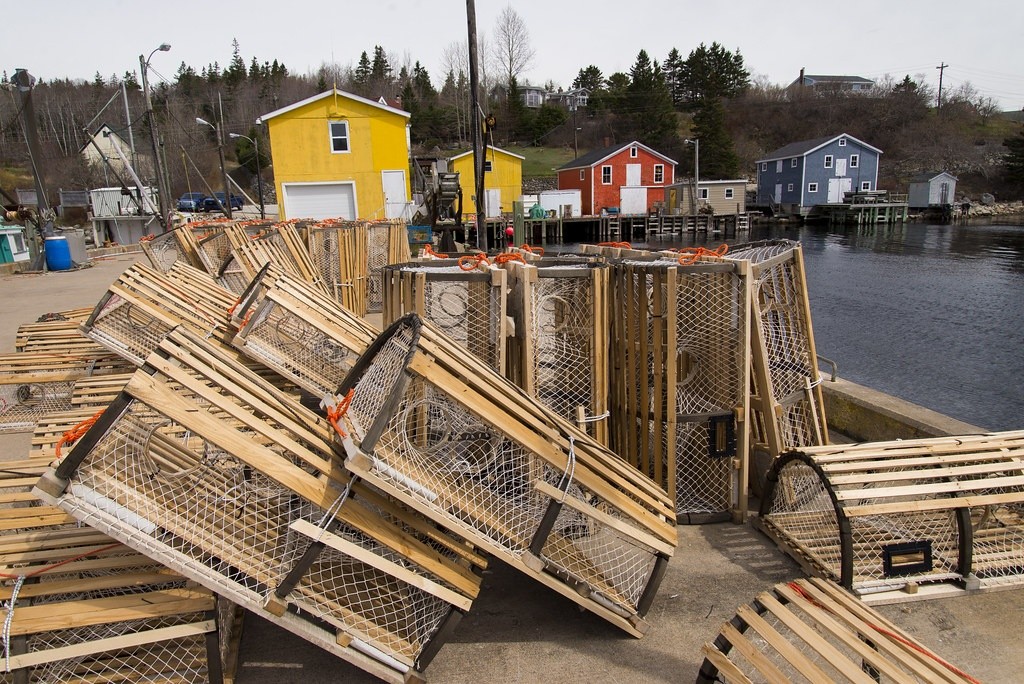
[139,44,172,219]
[195,117,232,219]
[229,133,264,219]
[684,138,698,213]
[574,127,582,158]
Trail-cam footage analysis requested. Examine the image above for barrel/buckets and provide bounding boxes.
[44,236,72,271]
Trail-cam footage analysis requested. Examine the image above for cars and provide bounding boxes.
[177,193,208,212]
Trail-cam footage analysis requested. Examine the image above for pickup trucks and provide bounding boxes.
[204,191,244,212]
